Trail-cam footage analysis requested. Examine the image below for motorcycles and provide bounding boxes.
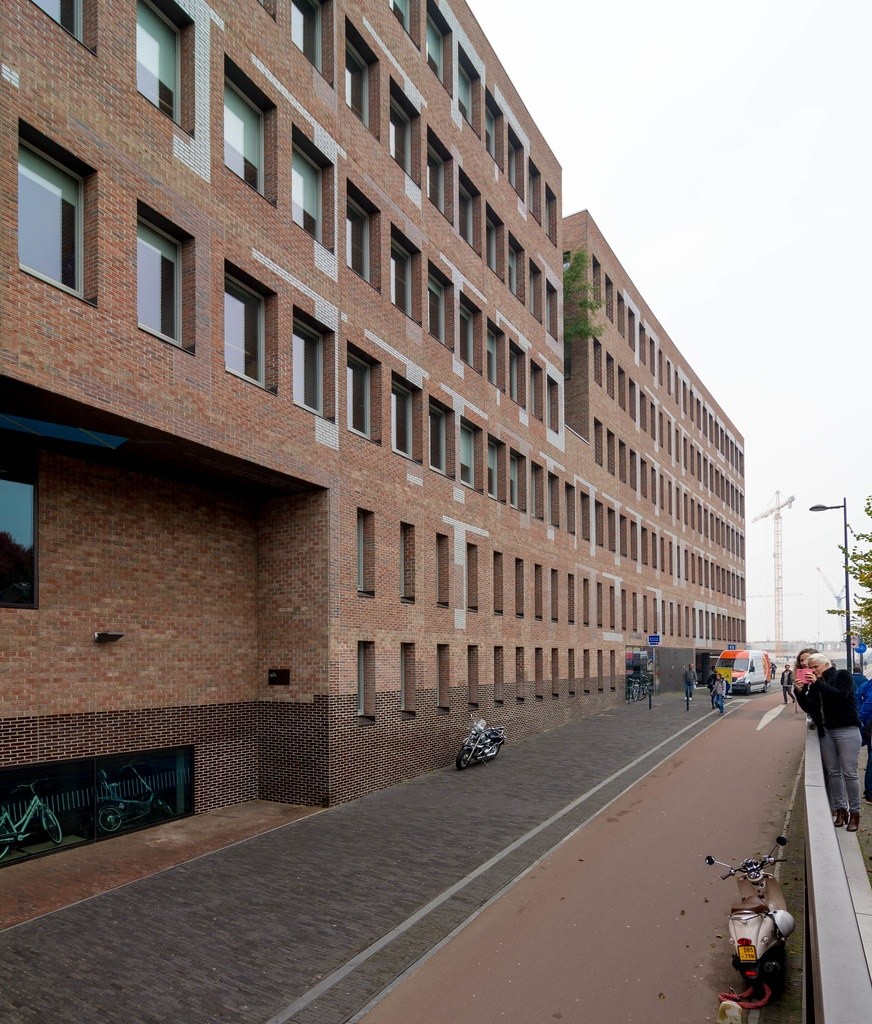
[455,713,507,770]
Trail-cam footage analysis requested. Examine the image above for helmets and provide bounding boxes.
[767,910,795,938]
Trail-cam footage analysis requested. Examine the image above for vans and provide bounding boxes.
[712,649,772,695]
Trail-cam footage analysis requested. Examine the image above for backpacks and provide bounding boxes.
[721,680,730,694]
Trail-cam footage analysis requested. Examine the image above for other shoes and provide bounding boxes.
[865,798,872,805]
[783,702,787,704]
[792,698,795,703]
[684,697,687,701]
[832,809,837,816]
[689,697,692,701]
[720,712,724,716]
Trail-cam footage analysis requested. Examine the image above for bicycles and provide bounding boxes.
[626,674,649,703]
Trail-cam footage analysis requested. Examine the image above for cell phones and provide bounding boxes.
[796,669,813,684]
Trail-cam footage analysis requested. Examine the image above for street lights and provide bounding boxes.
[809,496,852,677]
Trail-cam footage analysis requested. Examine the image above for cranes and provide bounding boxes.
[749,491,796,659]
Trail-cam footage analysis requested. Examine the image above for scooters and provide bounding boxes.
[703,836,795,1004]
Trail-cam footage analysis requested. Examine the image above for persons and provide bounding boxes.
[852,667,868,687]
[711,673,726,716]
[708,666,717,709]
[796,648,838,816]
[683,663,698,700]
[863,660,867,668]
[770,662,777,679]
[794,653,862,832]
[829,658,836,668]
[781,665,795,704]
[857,680,872,805]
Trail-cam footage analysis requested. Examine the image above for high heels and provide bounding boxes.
[834,808,849,827]
[846,811,859,831]
